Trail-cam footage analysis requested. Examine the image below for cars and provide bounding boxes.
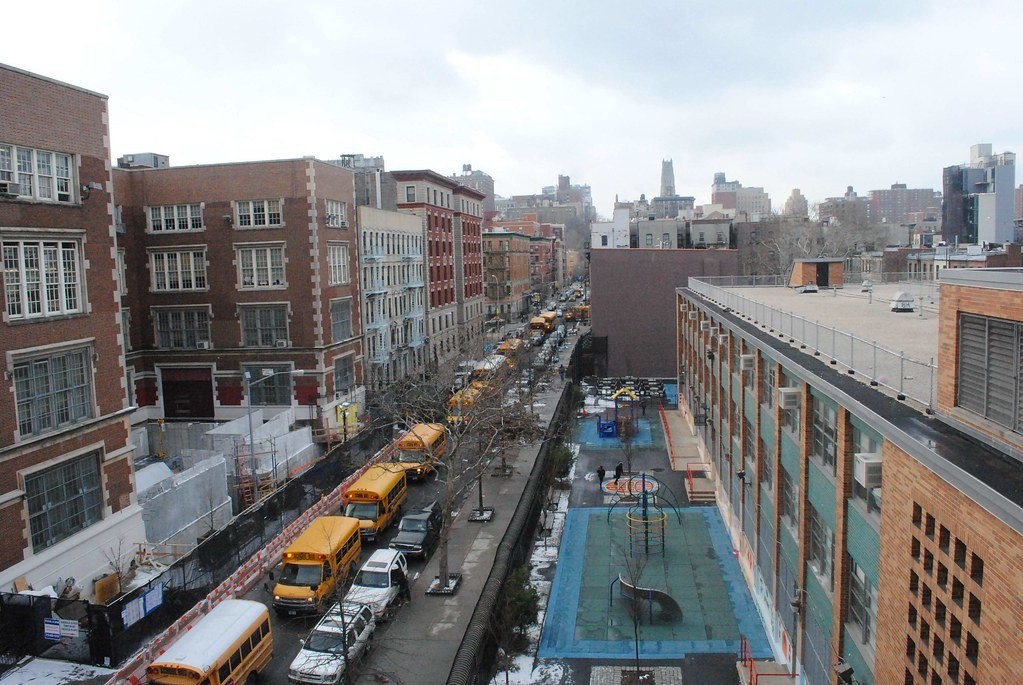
[447,375,465,393]
[454,360,476,381]
[494,281,590,411]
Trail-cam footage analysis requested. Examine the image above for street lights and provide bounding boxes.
[245,369,304,504]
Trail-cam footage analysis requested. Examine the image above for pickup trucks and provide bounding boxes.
[388,499,442,562]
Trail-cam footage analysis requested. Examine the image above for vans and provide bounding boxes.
[345,548,408,624]
[286,600,376,685]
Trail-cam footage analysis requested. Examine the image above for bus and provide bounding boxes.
[395,422,448,480]
[339,464,408,542]
[270,516,363,618]
[447,389,482,433]
[145,599,273,685]
[473,355,509,397]
